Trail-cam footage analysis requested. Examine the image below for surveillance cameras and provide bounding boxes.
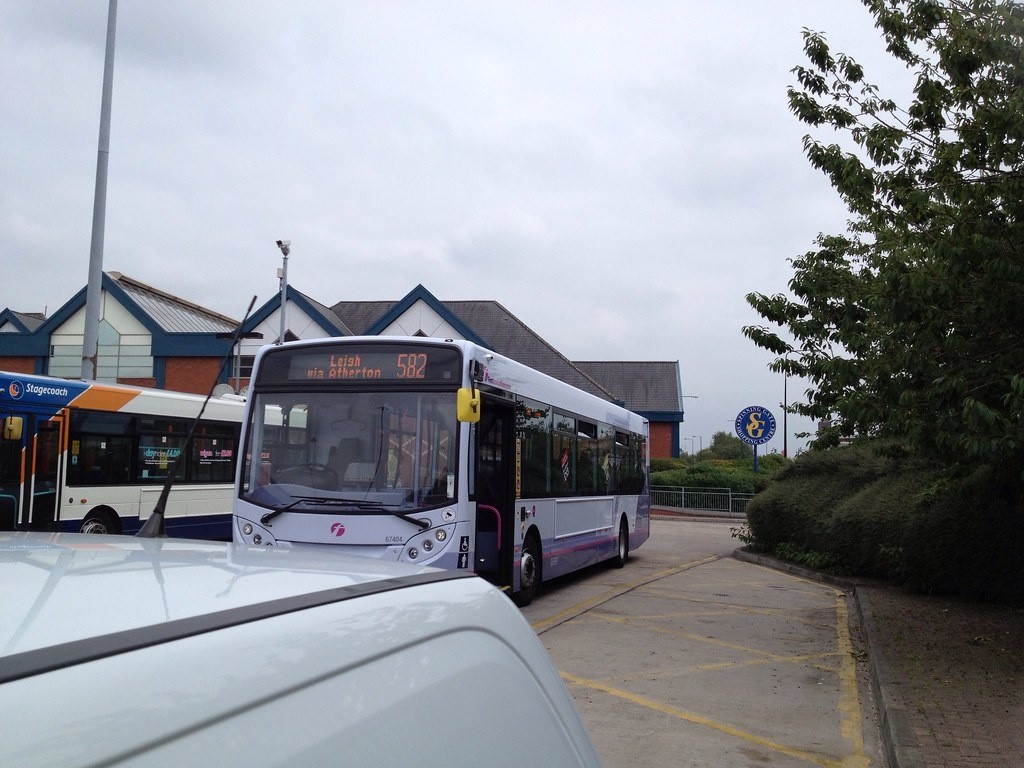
[276,240,291,248]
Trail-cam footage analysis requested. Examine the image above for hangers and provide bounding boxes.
[330,408,367,431]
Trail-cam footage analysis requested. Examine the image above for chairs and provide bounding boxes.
[326,438,361,484]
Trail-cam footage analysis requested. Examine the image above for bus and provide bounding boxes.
[231,336,650,609]
[0,371,307,543]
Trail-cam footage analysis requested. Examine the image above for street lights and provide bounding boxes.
[692,435,702,452]
[684,438,694,454]
[784,349,821,459]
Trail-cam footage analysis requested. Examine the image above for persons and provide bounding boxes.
[576,449,606,495]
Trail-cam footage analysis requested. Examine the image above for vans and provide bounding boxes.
[0,528,599,768]
